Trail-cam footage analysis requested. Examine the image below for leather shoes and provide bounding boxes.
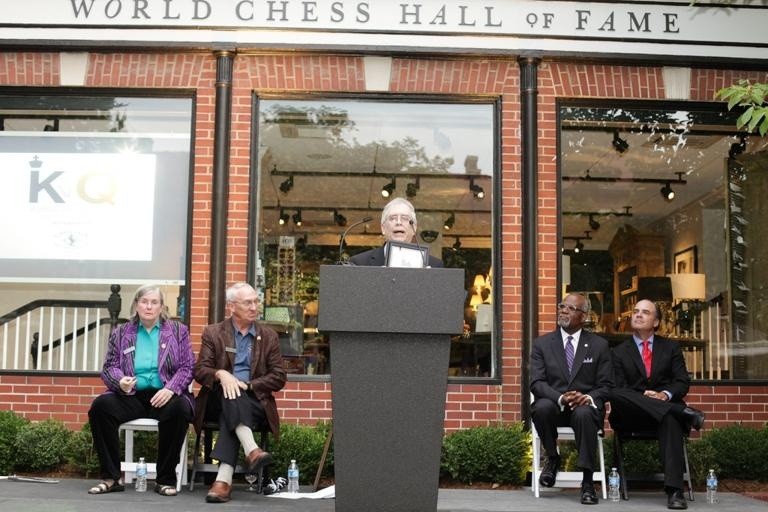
[245,447,271,473]
[580,480,599,504]
[667,488,687,508]
[539,454,563,486]
[205,481,234,501]
[682,406,705,431]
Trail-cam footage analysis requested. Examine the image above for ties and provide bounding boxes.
[565,336,574,370]
[642,341,652,379]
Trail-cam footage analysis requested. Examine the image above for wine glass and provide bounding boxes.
[244,474,257,491]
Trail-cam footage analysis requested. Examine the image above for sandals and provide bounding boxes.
[154,483,177,495]
[88,475,125,493]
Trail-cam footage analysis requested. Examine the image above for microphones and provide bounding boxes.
[409,220,432,268]
[334,216,373,266]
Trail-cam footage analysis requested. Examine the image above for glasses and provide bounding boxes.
[557,303,587,314]
[231,298,261,306]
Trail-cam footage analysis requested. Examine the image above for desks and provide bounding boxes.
[670,337,707,380]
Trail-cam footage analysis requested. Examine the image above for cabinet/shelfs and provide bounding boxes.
[610,233,667,327]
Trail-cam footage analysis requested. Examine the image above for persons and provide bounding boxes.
[528,290,616,505]
[87,284,196,497]
[347,196,444,267]
[195,281,288,503]
[610,296,705,510]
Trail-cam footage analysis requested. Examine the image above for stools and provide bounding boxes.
[611,426,695,503]
[188,419,272,495]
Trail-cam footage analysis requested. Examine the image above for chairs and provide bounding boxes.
[115,383,192,494]
[528,389,611,502]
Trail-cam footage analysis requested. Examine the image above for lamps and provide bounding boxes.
[289,232,592,257]
[273,204,633,231]
[666,274,705,336]
[561,124,766,160]
[267,144,686,201]
[635,275,672,337]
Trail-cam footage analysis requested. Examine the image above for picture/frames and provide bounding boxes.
[673,244,700,273]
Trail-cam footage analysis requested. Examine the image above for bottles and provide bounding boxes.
[134,455,148,494]
[706,469,717,505]
[606,467,621,503]
[286,457,301,493]
